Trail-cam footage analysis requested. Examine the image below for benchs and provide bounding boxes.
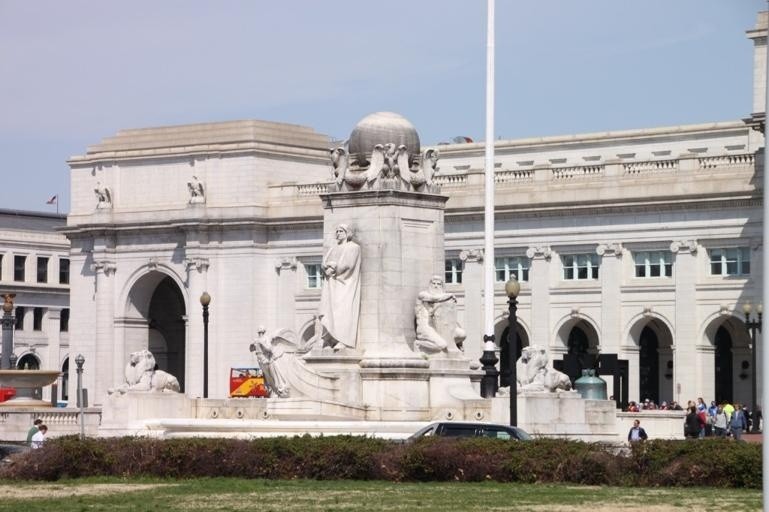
[0,440,46,458]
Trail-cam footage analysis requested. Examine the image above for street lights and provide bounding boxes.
[75,353,86,435]
[742,298,764,434]
[505,274,521,428]
[200,292,210,397]
[0,293,16,369]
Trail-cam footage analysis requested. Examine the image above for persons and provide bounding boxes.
[30,424,49,449]
[187,174,206,204]
[313,221,367,351]
[412,270,466,362]
[26,419,44,444]
[94,179,113,209]
[610,396,766,443]
[248,325,291,398]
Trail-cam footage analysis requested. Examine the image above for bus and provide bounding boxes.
[228,366,269,398]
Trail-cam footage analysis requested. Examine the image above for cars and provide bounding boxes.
[48,400,68,407]
[0,437,37,467]
[402,421,534,441]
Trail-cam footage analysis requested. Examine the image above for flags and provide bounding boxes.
[46,191,58,205]
